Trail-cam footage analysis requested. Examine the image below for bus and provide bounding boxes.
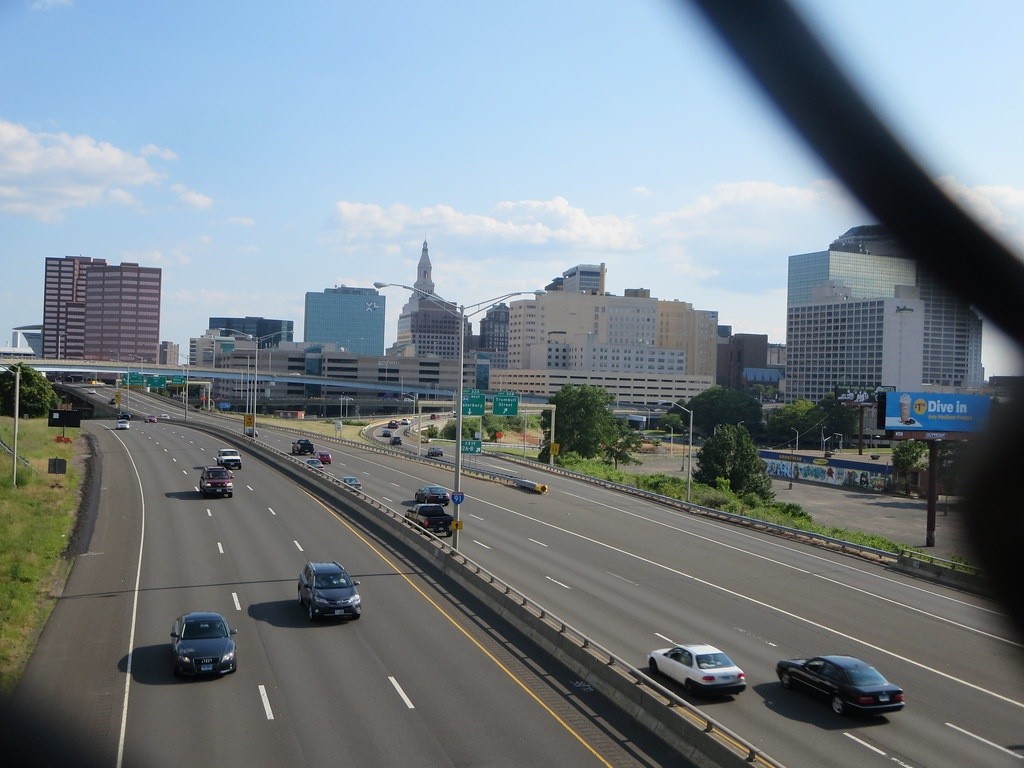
[649,643,748,695]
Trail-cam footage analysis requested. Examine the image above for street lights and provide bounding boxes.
[374,282,549,552]
[111,351,143,391]
[633,400,693,502]
[791,428,798,451]
[217,328,296,441]
[164,348,189,422]
[834,433,842,452]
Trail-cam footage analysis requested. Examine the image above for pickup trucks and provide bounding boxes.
[405,504,454,537]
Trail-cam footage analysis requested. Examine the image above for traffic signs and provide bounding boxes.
[461,393,486,454]
[493,395,518,416]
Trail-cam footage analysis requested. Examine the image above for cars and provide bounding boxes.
[88,389,170,430]
[414,486,450,506]
[775,655,905,714]
[246,413,443,495]
[217,449,242,470]
[172,611,238,676]
[199,466,234,498]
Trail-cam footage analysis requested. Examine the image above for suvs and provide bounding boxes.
[298,561,361,622]
